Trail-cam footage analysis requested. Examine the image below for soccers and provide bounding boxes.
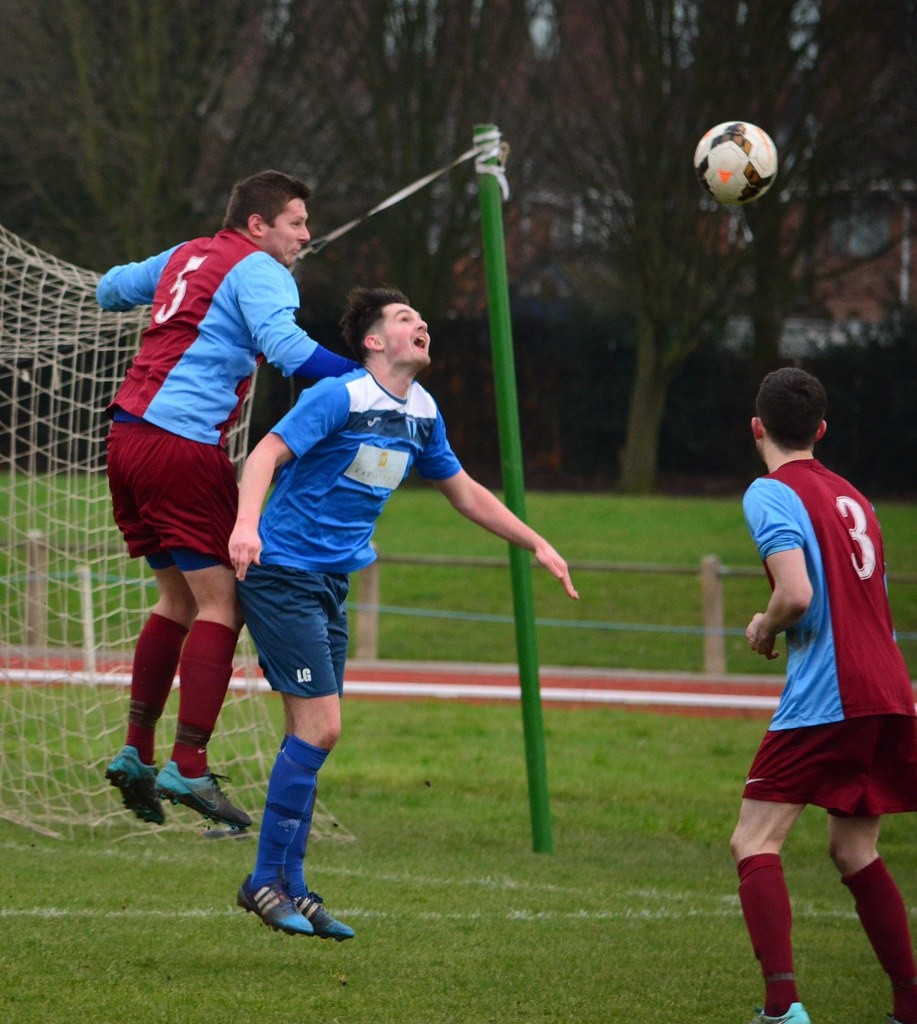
[693,120,780,206]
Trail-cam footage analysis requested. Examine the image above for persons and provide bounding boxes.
[97,172,361,831]
[226,289,583,942]
[728,367,917,1024]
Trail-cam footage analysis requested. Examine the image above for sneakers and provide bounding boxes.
[282,881,354,942]
[236,875,312,935]
[752,1002,809,1024]
[154,760,253,830]
[104,746,166,825]
